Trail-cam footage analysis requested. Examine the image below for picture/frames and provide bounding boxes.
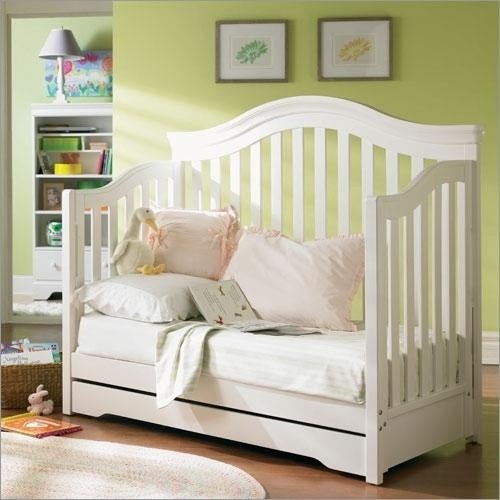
[213,17,290,84]
[316,15,394,81]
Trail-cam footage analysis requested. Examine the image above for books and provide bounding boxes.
[38,124,97,133]
[190,279,319,336]
[97,148,111,175]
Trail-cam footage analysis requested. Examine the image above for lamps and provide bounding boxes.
[39,25,83,104]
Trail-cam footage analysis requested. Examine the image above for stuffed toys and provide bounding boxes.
[25,383,53,414]
[111,205,164,274]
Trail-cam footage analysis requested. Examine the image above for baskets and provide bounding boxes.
[1,352,62,409]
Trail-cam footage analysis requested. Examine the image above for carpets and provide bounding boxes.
[1,431,267,500]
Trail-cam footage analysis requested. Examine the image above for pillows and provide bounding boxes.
[147,207,237,276]
[70,274,217,324]
[218,223,364,333]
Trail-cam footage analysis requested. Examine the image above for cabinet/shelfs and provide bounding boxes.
[29,103,112,256]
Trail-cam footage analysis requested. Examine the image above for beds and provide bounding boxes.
[59,94,489,485]
